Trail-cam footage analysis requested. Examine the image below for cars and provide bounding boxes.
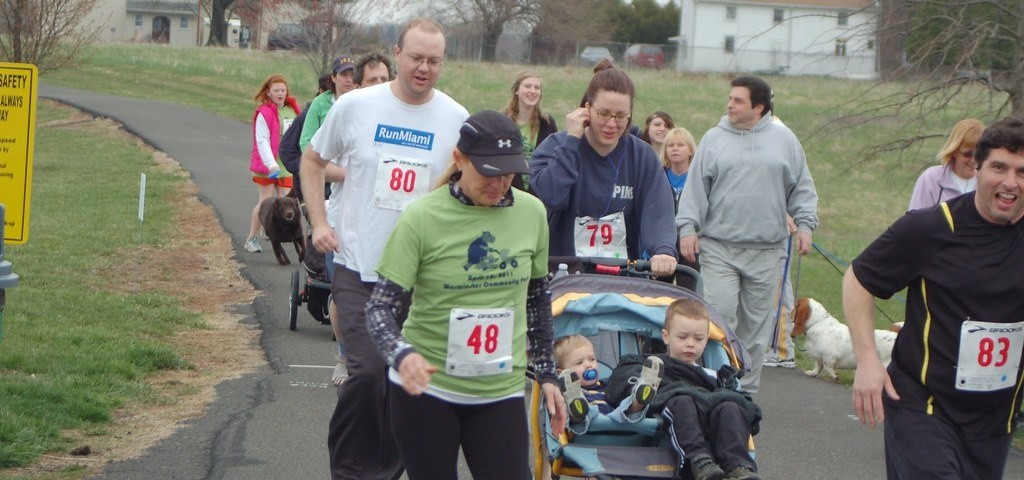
[572,46,616,66]
[622,44,665,69]
[266,22,317,50]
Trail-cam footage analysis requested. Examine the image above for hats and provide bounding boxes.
[456,110,530,176]
[333,55,356,72]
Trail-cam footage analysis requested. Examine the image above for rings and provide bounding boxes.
[579,112,583,116]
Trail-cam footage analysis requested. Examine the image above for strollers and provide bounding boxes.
[524,254,762,480]
[287,233,338,344]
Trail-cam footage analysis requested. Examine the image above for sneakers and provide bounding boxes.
[763,354,795,368]
[724,466,760,480]
[628,356,664,406]
[261,229,270,240]
[557,369,589,419]
[245,236,262,252]
[691,454,724,480]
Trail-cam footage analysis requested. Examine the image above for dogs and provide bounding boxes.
[258,196,305,265]
[788,296,905,382]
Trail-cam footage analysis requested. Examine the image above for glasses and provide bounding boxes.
[958,147,976,157]
[589,100,631,121]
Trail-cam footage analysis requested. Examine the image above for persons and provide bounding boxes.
[909,118,986,211]
[762,89,795,368]
[639,127,700,289]
[364,111,568,480]
[552,335,664,435]
[529,58,680,282]
[278,74,333,201]
[605,298,763,480]
[640,111,675,156]
[498,69,558,191]
[326,53,393,386]
[842,115,1024,480]
[299,56,358,153]
[299,16,470,480]
[674,75,819,395]
[244,76,302,252]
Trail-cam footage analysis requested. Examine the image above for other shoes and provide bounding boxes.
[331,355,348,386]
